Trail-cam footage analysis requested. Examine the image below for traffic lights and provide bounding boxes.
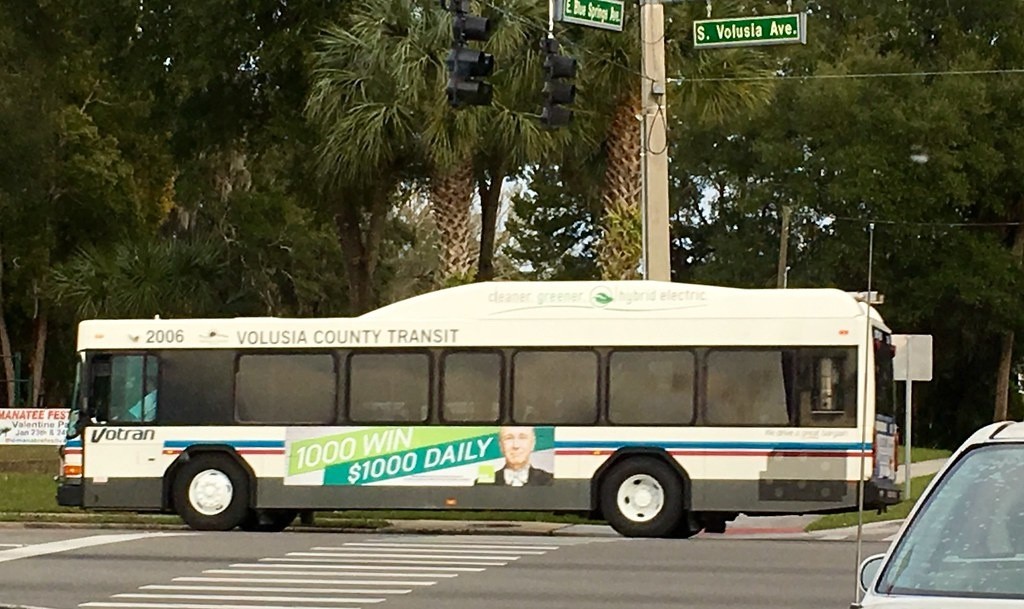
[540,54,578,127]
[444,14,494,111]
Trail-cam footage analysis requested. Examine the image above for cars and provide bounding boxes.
[858,421,1024,609]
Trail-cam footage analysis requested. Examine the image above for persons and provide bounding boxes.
[474,426,554,486]
[118,375,157,422]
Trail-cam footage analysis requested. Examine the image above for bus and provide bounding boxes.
[53,279,905,538]
